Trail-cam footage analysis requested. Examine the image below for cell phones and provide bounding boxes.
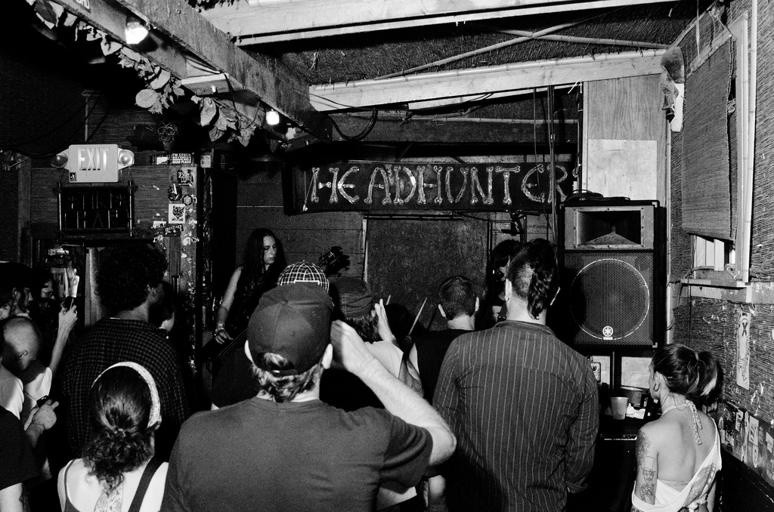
[62,297,75,313]
[36,394,51,408]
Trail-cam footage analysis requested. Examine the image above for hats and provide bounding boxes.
[247,282,333,377]
[331,279,373,317]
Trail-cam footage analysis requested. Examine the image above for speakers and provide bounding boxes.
[554,198,667,349]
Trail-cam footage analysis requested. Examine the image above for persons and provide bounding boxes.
[0,227,600,512]
[630,342,723,511]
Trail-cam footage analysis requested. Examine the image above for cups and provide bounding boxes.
[611,397,628,420]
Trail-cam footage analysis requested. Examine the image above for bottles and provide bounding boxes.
[62,275,81,315]
